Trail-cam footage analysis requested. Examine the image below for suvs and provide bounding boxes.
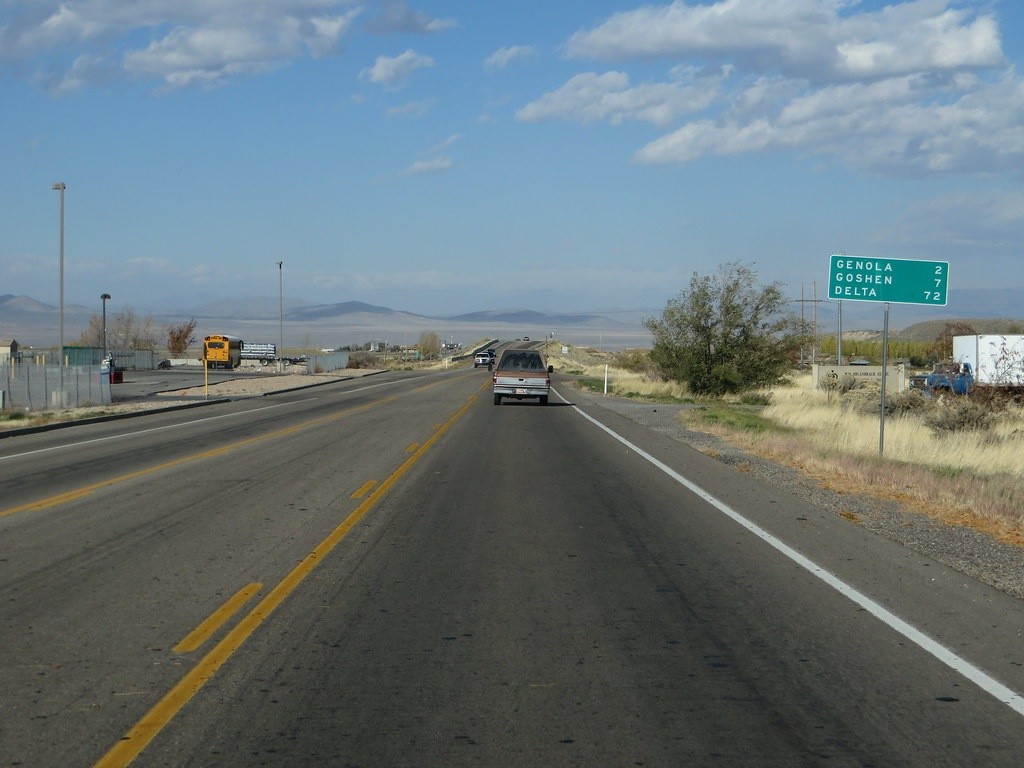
[473,353,492,368]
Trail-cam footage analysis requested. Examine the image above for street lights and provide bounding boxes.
[101,294,111,359]
[53,181,68,364]
[277,261,287,373]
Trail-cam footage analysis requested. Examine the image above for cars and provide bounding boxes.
[493,349,554,407]
[524,337,529,341]
[482,352,495,364]
[488,349,496,357]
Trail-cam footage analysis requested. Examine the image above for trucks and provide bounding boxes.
[923,334,1023,397]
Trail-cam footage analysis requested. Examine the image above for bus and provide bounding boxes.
[203,335,244,369]
